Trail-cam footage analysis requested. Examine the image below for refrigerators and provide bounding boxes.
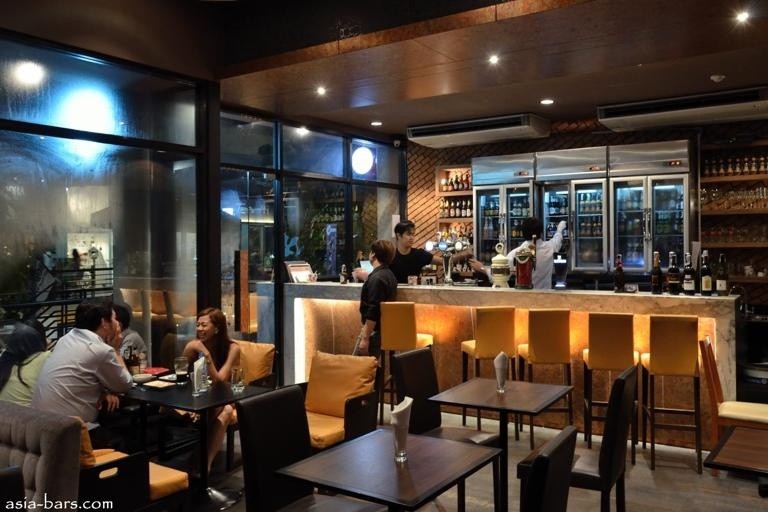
[607,139,696,274]
[471,153,535,267]
[534,146,608,276]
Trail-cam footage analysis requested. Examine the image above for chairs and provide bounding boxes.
[0,465,27,510]
[78,453,150,511]
[121,286,257,365]
[700,335,767,476]
[68,414,188,512]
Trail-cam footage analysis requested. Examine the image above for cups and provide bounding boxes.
[494,367,508,392]
[408,276,418,286]
[231,368,245,393]
[553,255,568,290]
[173,357,189,376]
[390,424,410,463]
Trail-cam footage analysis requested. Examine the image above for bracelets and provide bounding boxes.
[479,265,485,272]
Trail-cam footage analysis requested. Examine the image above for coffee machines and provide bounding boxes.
[513,233,538,289]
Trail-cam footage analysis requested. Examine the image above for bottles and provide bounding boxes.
[656,211,683,234]
[441,173,471,192]
[619,216,626,233]
[667,251,681,294]
[340,264,347,284]
[579,192,601,213]
[546,220,567,238]
[658,186,683,210]
[681,253,698,296]
[715,254,728,296]
[700,249,713,296]
[614,253,623,292]
[651,250,662,293]
[624,238,644,265]
[549,194,568,215]
[439,199,472,218]
[511,197,529,239]
[482,201,499,262]
[580,217,602,238]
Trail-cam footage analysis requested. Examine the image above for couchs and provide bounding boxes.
[0,399,81,512]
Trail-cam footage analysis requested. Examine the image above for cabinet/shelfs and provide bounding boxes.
[240,192,305,279]
[698,125,768,316]
[316,180,362,252]
[436,161,472,247]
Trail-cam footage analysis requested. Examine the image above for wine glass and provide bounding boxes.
[699,186,766,210]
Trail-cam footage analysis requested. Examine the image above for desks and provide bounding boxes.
[702,427,768,476]
[101,369,274,511]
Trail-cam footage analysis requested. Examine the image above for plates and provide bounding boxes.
[143,379,176,389]
[159,371,178,382]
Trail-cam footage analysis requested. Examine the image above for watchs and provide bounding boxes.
[207,358,213,365]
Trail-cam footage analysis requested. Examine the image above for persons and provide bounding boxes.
[351,219,475,283]
[357,239,398,358]
[107,303,148,363]
[29,296,134,451]
[146,307,243,495]
[0,316,52,407]
[467,215,567,289]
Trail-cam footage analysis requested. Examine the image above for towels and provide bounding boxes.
[192,355,205,394]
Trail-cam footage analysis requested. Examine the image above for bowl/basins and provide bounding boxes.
[132,373,153,383]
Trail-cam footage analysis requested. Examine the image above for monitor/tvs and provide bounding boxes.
[360,259,375,275]
